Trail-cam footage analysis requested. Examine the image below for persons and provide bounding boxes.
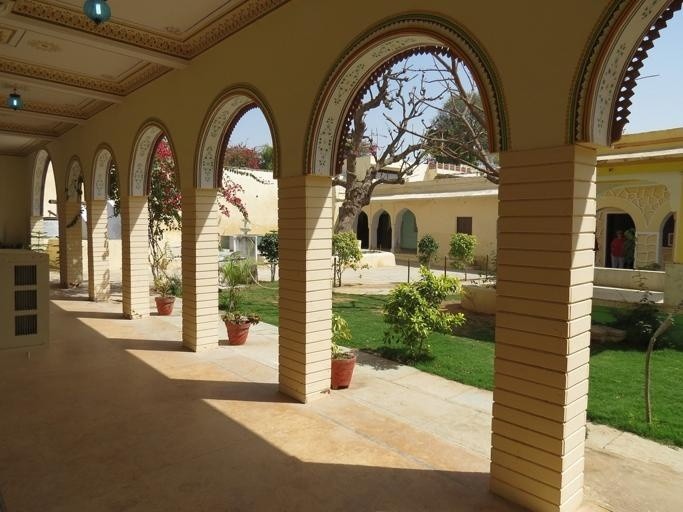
[622,228,635,268]
[609,230,628,269]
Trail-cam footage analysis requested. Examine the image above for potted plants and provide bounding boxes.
[331,312,356,389]
[221,309,262,345]
[154,276,175,315]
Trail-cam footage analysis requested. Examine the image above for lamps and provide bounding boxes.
[84,0,111,25]
[8,88,22,111]
